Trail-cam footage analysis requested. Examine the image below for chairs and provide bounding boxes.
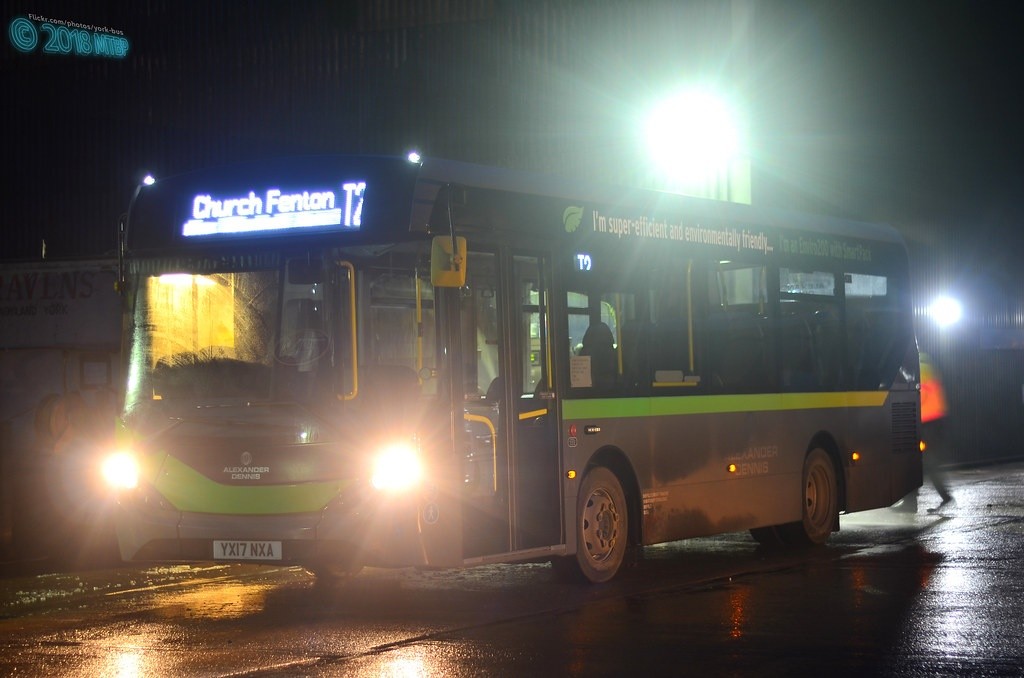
[579,292,889,397]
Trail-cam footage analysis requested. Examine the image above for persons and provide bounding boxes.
[891,350,961,515]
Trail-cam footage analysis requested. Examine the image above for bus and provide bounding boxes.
[113,152,926,586]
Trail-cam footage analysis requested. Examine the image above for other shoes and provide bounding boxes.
[926,495,955,513]
[890,496,917,514]
